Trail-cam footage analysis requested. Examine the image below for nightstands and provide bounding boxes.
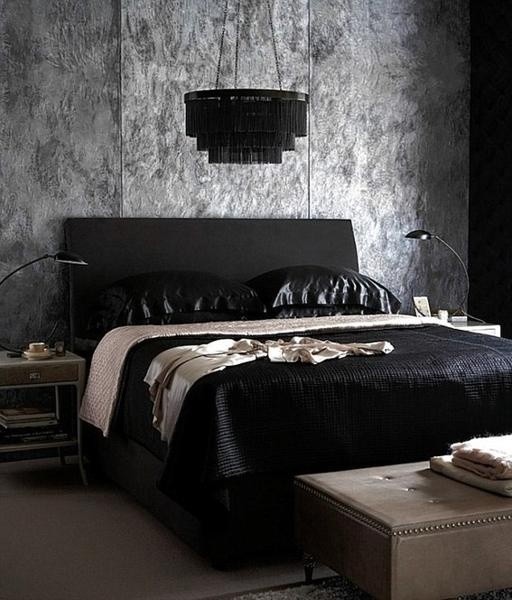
[0,347,94,489]
[423,306,503,344]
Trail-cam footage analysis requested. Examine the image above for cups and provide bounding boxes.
[29,343,49,353]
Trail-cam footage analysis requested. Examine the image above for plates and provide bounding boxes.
[22,351,56,358]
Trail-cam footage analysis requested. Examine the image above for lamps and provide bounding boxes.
[404,230,470,324]
[181,0,310,167]
[0,251,89,290]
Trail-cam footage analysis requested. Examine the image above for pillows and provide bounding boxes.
[97,268,262,318]
[241,255,412,314]
[89,306,251,335]
[264,305,376,321]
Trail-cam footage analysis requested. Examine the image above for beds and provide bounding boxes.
[60,212,512,570]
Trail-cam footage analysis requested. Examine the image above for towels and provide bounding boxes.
[428,433,512,497]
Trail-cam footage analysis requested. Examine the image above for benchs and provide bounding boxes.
[292,461,512,600]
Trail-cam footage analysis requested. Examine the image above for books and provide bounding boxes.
[0,407,58,429]
[448,314,468,322]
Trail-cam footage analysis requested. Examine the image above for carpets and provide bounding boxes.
[194,575,511,600]
